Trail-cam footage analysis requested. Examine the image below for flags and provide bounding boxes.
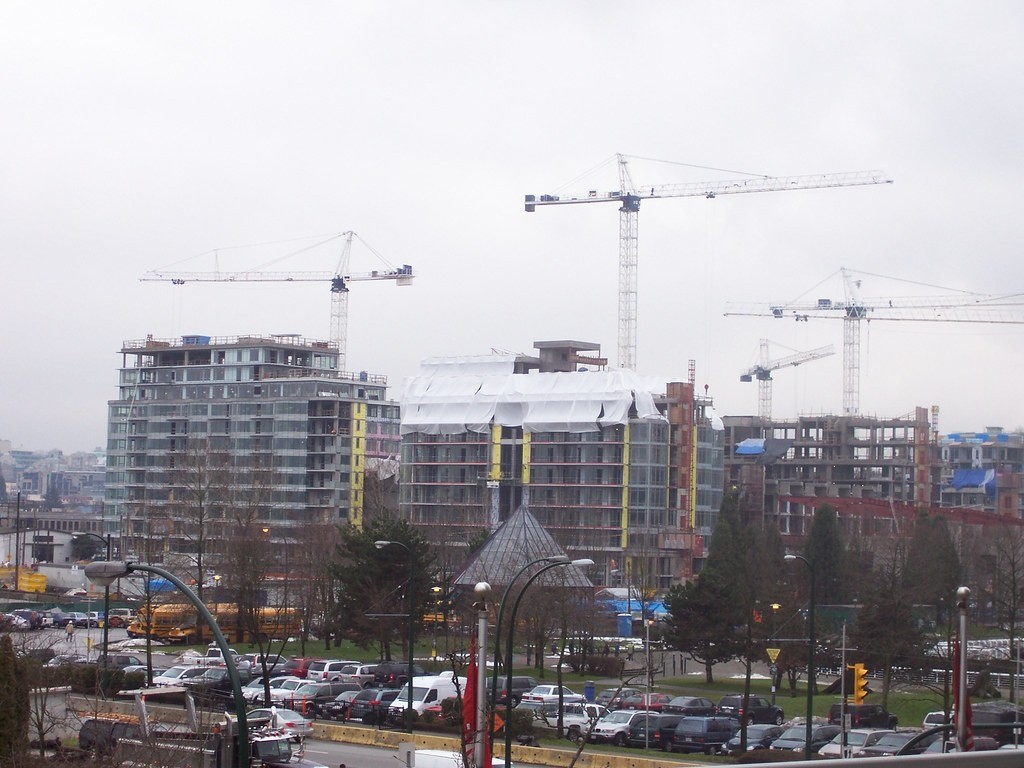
[953,629,975,751]
[462,638,491,768]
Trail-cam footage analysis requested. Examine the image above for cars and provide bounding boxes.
[0,600,1023,767]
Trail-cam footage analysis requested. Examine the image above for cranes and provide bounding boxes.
[525,150,896,370]
[139,231,416,374]
[722,267,1022,413]
[739,335,834,422]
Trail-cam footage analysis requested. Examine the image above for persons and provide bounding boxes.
[614,641,619,657]
[551,641,558,656]
[65,620,74,642]
[628,642,634,661]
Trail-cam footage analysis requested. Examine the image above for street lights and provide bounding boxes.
[374,540,416,734]
[84,560,251,766]
[954,586,972,750]
[488,553,570,766]
[472,581,491,767]
[508,559,595,768]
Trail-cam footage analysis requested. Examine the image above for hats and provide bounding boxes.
[69,620,72,624]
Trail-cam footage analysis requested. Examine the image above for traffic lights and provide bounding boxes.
[854,663,869,706]
